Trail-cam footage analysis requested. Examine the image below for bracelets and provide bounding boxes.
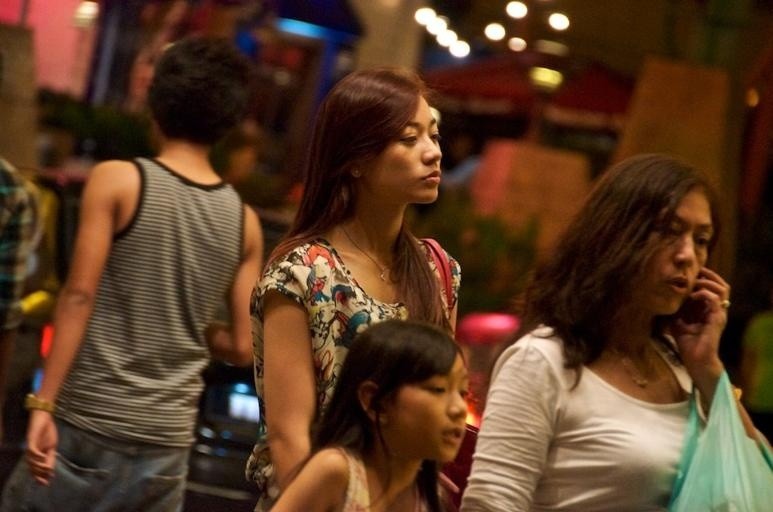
[604,334,655,387]
[23,393,53,412]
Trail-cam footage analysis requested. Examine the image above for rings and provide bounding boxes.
[720,300,730,307]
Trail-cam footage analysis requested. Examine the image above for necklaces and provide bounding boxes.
[338,221,408,288]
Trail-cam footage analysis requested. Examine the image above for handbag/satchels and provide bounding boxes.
[665,368,772,512]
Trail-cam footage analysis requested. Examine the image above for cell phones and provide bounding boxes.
[684,275,707,325]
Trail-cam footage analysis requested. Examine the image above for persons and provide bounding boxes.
[253,66,460,512]
[258,314,470,512]
[0,31,266,511]
[738,310,772,413]
[456,151,772,512]
